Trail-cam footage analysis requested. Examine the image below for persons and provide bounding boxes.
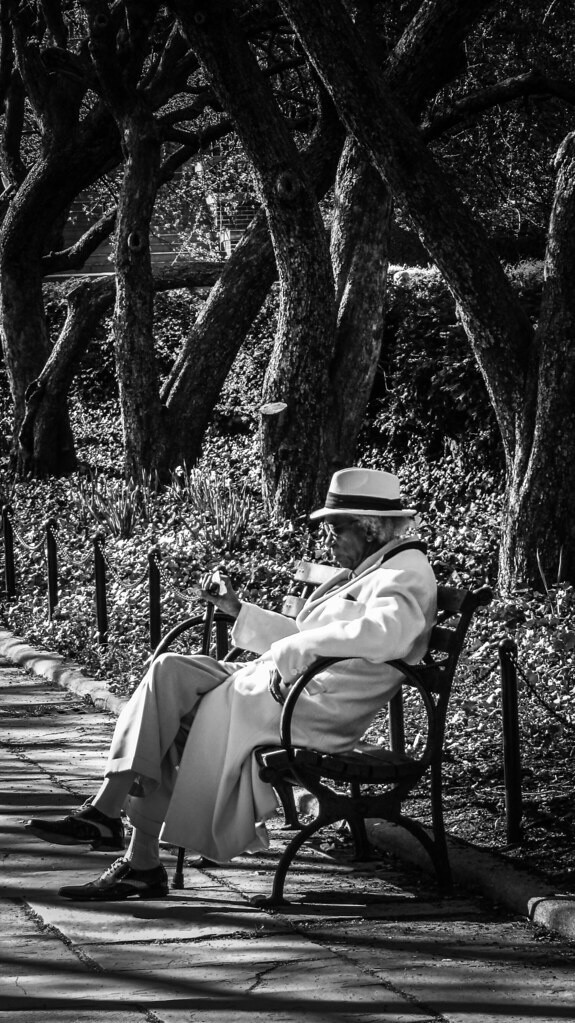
[25,467,438,902]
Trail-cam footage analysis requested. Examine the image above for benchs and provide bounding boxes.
[150,562,493,910]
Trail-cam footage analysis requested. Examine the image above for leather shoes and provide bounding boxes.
[58,857,170,900]
[24,795,125,852]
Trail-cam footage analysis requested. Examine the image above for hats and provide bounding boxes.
[310,467,417,520]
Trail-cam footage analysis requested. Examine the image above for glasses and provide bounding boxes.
[323,519,359,538]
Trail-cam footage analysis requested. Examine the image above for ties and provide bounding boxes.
[321,573,357,597]
